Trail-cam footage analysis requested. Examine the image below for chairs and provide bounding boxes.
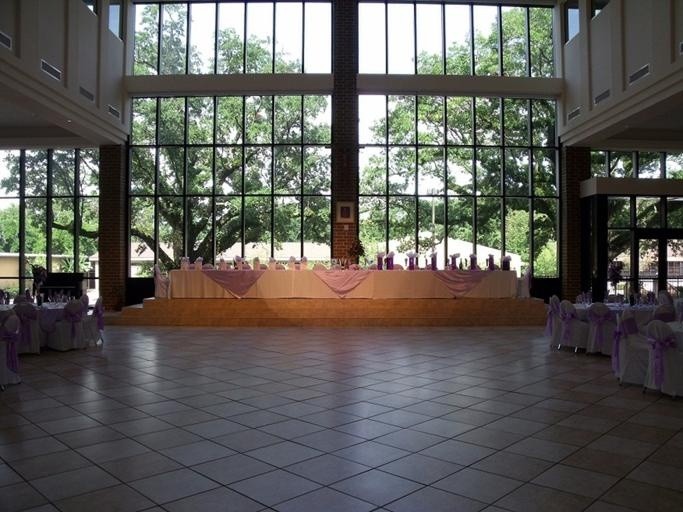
[0,285,103,391]
[543,286,683,402]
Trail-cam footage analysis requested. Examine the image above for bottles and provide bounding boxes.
[180,254,510,270]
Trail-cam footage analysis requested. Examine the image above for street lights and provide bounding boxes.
[424,187,442,253]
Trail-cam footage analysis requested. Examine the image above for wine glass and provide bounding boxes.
[573,293,656,308]
[3,290,75,306]
